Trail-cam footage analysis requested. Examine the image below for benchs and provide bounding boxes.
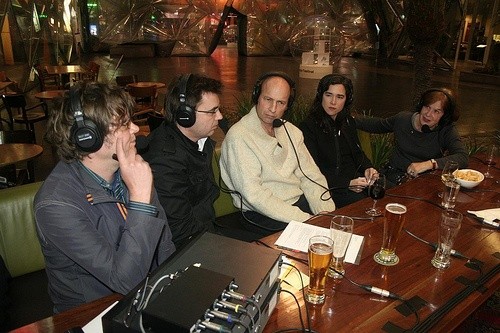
[1,125,395,320]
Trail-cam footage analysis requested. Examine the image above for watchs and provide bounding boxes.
[430,159,436,170]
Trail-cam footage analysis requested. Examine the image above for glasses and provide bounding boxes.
[111,117,133,129]
[195,104,221,117]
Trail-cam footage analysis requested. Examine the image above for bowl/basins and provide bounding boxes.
[452,169,484,189]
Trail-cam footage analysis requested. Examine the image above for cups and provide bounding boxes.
[484,144,498,179]
[304,235,334,304]
[328,214,353,279]
[442,177,460,210]
[373,203,407,266]
[430,209,462,268]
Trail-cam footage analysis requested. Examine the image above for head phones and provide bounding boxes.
[251,72,297,114]
[176,73,196,128]
[70,89,104,153]
[415,87,456,127]
[318,76,353,105]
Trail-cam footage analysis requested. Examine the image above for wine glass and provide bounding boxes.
[437,161,459,198]
[365,174,386,216]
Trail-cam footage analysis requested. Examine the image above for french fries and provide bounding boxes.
[455,170,482,181]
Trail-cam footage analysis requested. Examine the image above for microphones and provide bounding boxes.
[272,115,289,128]
[421,124,436,133]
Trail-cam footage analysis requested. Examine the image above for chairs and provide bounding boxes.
[1,94,50,144]
[125,82,156,110]
[35,65,61,92]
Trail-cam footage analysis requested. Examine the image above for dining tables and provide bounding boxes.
[0,62,500,333]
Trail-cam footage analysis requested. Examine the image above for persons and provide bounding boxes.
[300,74,380,209]
[218,76,335,225]
[136,74,223,248]
[349,87,469,190]
[33,84,176,312]
